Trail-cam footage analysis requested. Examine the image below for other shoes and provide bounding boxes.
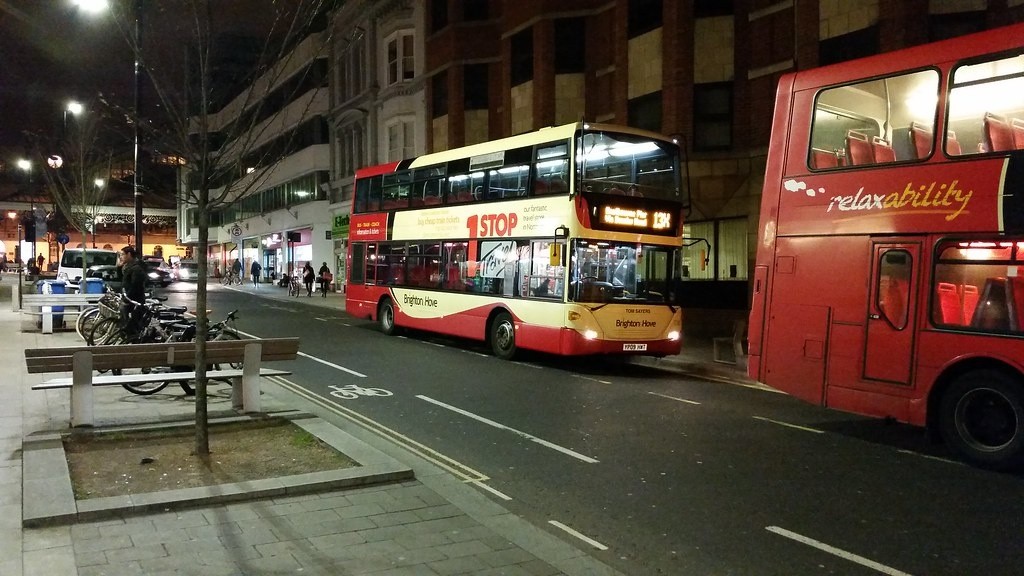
[321,292,324,297]
[307,291,311,297]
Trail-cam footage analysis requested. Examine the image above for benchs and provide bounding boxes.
[21,293,150,335]
[712,318,747,365]
[6,262,26,274]
[24,337,300,428]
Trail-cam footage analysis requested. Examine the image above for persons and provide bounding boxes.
[319,262,330,298]
[115,246,147,344]
[232,259,244,277]
[3,253,8,271]
[303,262,315,297]
[250,260,261,286]
[37,253,43,271]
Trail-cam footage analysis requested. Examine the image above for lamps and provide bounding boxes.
[285,205,299,219]
[235,218,249,231]
[259,212,271,225]
[220,224,231,235]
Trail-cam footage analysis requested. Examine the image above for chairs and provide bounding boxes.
[970,276,1009,329]
[457,187,474,201]
[627,190,644,197]
[957,284,979,325]
[934,282,962,324]
[907,121,933,159]
[1004,276,1024,331]
[947,130,962,155]
[870,135,897,163]
[812,147,839,169]
[608,188,626,196]
[548,174,568,193]
[447,192,457,204]
[1008,117,1024,149]
[367,192,442,211]
[981,112,1017,153]
[844,129,875,166]
[535,178,551,194]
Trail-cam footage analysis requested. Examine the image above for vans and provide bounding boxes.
[56,248,199,288]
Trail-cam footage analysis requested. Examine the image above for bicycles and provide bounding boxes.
[75,283,244,395]
[220,271,243,286]
[288,276,300,298]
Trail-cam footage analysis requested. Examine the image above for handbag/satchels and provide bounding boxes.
[323,272,332,281]
[303,267,310,278]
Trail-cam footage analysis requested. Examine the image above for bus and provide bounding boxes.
[746,19,1023,478]
[345,115,712,362]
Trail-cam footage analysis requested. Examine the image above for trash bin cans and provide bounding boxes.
[78,277,104,310]
[37,280,67,329]
